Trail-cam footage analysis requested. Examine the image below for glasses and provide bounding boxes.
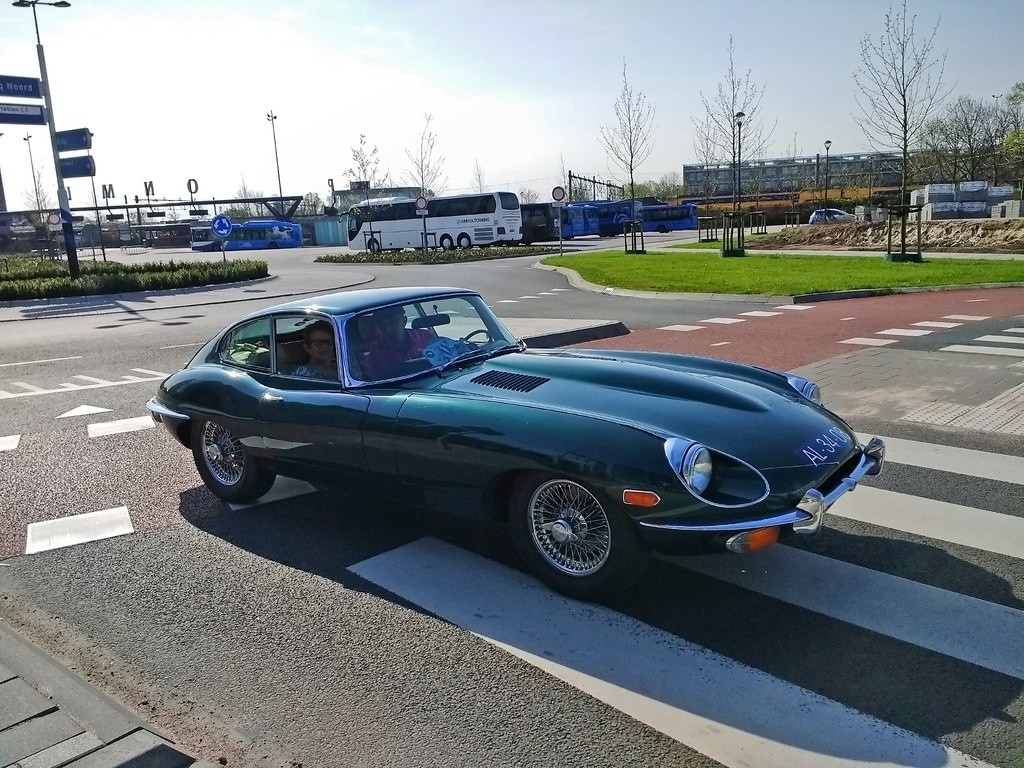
[306,339,335,347]
[378,314,403,327]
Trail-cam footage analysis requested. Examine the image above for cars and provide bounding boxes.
[810,209,856,223]
[143,285,888,599]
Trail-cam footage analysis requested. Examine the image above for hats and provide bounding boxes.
[376,306,406,319]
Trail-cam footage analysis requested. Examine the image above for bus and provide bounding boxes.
[189,226,218,251]
[638,204,699,235]
[337,191,523,249]
[222,221,303,251]
[519,202,599,246]
[567,200,642,239]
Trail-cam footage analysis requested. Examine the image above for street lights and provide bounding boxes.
[10,0,83,279]
[736,112,746,214]
[265,109,287,216]
[823,140,833,209]
[21,130,42,225]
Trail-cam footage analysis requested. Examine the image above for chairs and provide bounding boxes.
[278,340,311,366]
[358,317,378,366]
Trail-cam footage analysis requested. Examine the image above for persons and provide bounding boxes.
[292,321,360,382]
[364,306,438,379]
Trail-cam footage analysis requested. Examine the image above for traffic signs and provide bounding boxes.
[59,156,96,179]
[0,103,45,125]
[54,128,92,154]
[0,75,42,99]
